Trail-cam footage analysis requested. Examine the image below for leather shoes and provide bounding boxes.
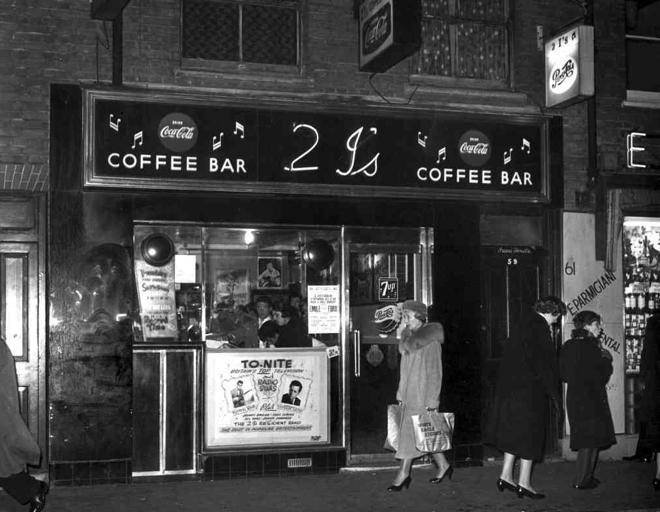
[572,484,584,489]
[622,455,651,464]
[30,480,49,512]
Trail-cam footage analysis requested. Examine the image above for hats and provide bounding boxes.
[403,299,426,314]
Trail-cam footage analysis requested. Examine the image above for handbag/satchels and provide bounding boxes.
[383,402,404,452]
[411,408,455,452]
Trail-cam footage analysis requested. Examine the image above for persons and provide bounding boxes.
[387,300,453,492]
[282,380,302,406]
[485,296,568,499]
[231,381,245,408]
[620,313,660,491]
[559,310,617,489]
[177,296,308,347]
[0,338,48,512]
[258,262,280,288]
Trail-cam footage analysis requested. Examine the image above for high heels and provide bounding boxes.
[429,465,454,484]
[516,485,544,499]
[652,478,660,492]
[387,476,411,493]
[497,478,519,492]
